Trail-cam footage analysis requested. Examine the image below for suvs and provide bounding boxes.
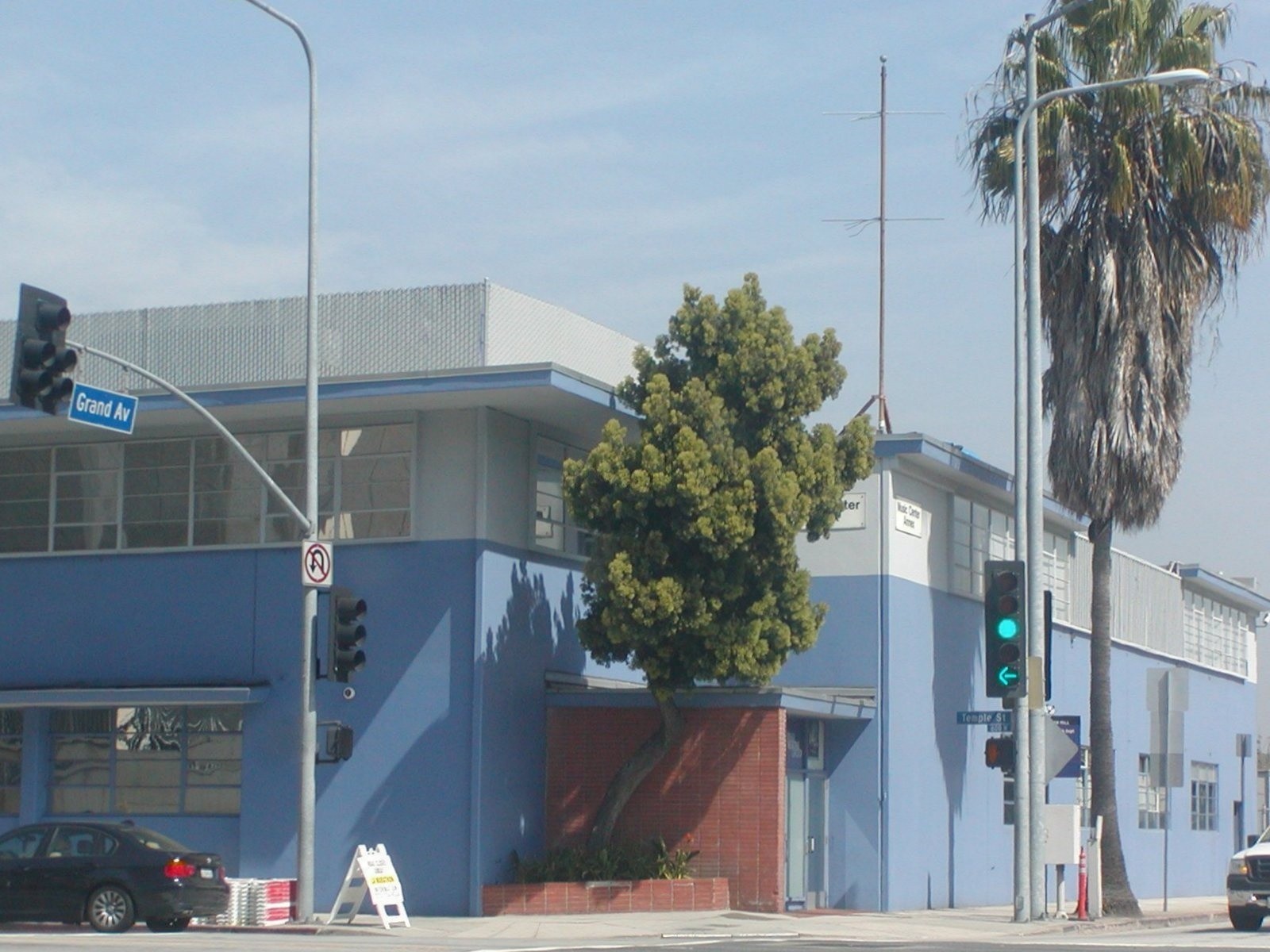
[1226,821,1270,932]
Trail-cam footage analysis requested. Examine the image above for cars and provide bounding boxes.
[0,820,230,933]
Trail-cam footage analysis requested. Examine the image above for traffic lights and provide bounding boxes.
[325,726,354,759]
[985,740,1015,768]
[9,284,77,416]
[984,561,1026,695]
[329,587,367,684]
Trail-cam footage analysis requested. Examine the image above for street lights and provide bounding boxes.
[1009,68,1212,921]
